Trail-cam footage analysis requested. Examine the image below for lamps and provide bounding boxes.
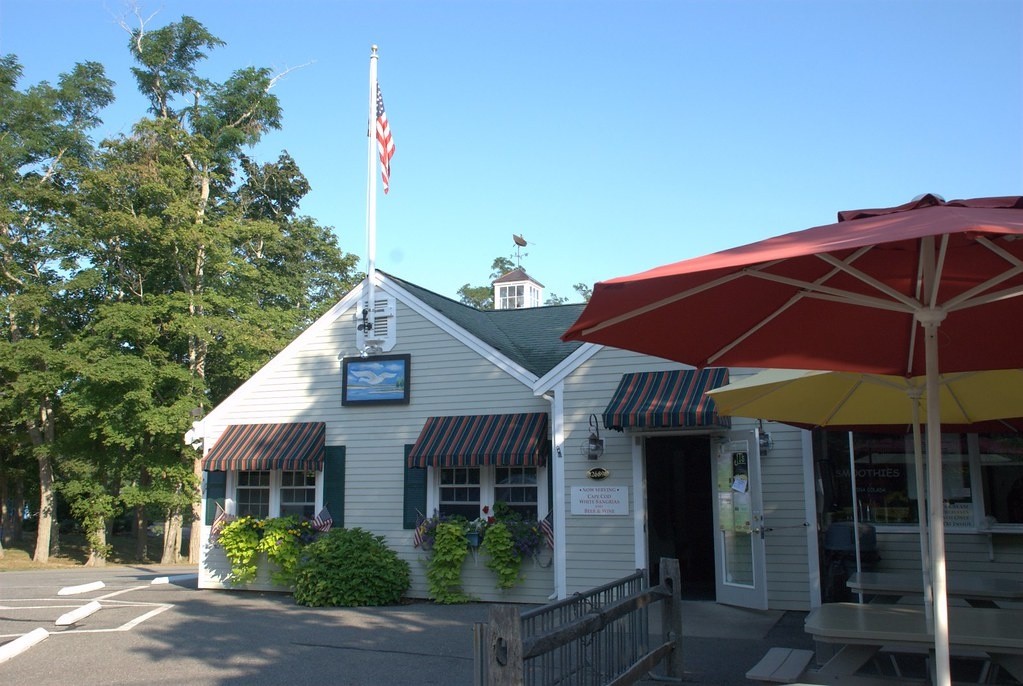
[580,414,604,459]
[753,418,775,455]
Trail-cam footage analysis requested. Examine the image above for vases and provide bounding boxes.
[466,533,480,547]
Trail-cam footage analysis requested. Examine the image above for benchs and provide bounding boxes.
[744,595,1022,686]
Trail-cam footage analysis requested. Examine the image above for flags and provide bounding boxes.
[537,508,554,551]
[367,58,396,195]
[412,510,431,549]
[209,503,228,544]
[300,506,333,534]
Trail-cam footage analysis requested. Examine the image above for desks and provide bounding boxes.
[804,607,1023,686]
[846,569,1022,606]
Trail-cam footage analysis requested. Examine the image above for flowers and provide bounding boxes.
[471,506,496,534]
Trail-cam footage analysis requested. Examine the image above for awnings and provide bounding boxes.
[600,367,734,428]
[407,412,549,468]
[201,421,326,471]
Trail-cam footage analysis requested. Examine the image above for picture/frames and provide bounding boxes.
[341,352,410,406]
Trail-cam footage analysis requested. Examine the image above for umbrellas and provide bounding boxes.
[562,192,1023,686]
